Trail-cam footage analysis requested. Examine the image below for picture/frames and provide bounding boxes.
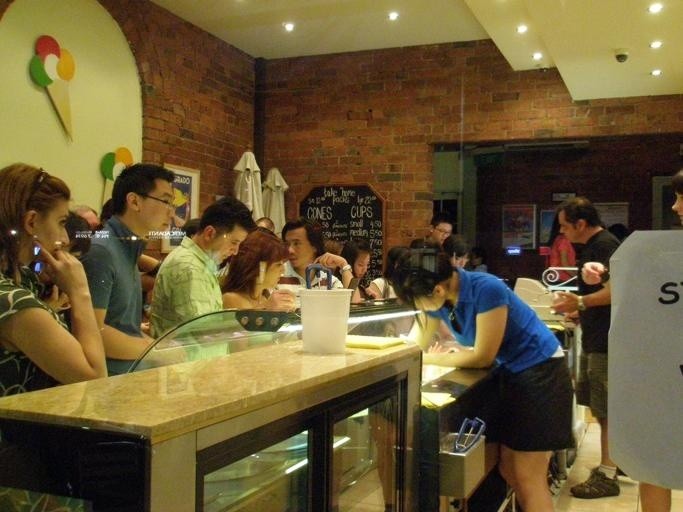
[539,209,557,243]
[161,162,201,253]
[502,204,537,251]
[592,202,630,232]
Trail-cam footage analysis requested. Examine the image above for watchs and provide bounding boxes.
[575,295,587,311]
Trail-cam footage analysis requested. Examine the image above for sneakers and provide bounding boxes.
[591,465,640,486]
[570,468,620,499]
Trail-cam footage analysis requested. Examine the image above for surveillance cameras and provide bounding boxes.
[614,51,629,63]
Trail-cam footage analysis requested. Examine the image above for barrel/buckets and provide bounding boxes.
[297,290,352,354]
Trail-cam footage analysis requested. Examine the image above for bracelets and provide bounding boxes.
[339,263,353,275]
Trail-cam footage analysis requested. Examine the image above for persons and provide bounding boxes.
[1,162,144,511]
[391,246,576,512]
[641,169,683,512]
[147,195,253,339]
[91,199,114,230]
[545,211,579,286]
[40,209,92,260]
[441,233,468,269]
[468,246,488,273]
[255,217,275,232]
[548,194,623,498]
[139,255,161,292]
[78,163,189,376]
[278,215,362,303]
[337,237,379,302]
[369,392,402,512]
[367,246,411,300]
[409,211,453,250]
[222,228,295,310]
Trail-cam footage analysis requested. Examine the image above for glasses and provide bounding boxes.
[433,228,452,237]
[27,168,52,210]
[134,193,178,211]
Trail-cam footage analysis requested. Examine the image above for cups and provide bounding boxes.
[539,246,551,255]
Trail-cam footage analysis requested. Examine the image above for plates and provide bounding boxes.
[261,433,307,459]
[201,456,273,484]
[367,299,402,305]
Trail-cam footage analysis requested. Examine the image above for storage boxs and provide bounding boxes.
[438,430,487,499]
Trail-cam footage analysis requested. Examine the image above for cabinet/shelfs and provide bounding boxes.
[0,334,422,512]
[129,296,435,373]
